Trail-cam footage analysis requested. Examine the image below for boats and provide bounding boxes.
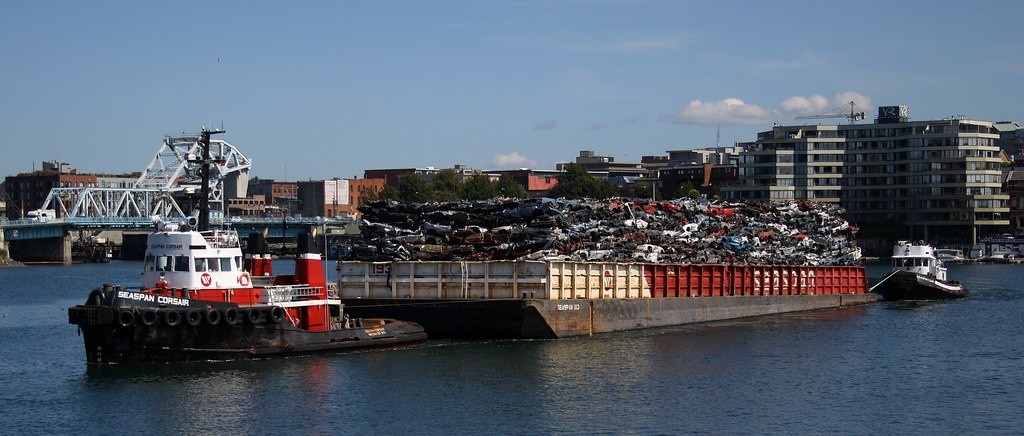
[249,252,880,342]
[65,216,430,370]
[882,240,969,302]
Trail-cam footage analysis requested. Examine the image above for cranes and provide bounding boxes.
[794,99,868,125]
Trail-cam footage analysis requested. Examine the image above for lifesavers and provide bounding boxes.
[184,309,201,327]
[204,308,221,326]
[246,307,262,325]
[88,289,106,307]
[269,307,285,324]
[239,273,250,286]
[222,306,240,325]
[164,309,181,327]
[117,310,135,328]
[141,308,158,326]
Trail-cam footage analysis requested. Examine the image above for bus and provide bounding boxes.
[936,248,964,260]
